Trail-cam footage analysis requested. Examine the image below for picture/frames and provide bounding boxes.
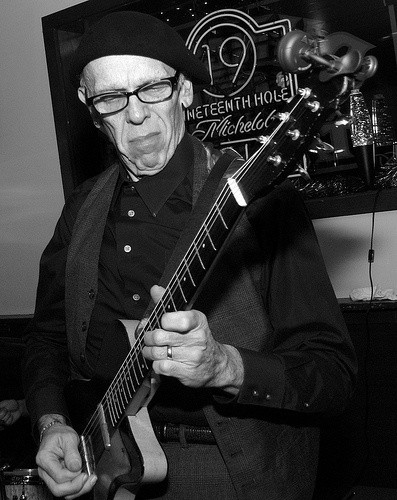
[40,1,396,220]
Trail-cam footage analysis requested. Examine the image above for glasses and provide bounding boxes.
[84,70,179,117]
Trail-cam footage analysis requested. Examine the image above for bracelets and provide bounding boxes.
[39,419,64,443]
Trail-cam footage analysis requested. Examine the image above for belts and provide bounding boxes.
[151,424,217,445]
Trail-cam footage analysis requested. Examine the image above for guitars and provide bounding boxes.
[71,28,379,500]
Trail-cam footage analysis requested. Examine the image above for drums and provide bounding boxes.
[0,468,48,500]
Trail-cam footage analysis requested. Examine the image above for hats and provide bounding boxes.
[75,12,213,85]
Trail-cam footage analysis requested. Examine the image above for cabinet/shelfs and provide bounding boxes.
[0,296,397,500]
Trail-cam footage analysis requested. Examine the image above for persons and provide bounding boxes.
[0,398,31,430]
[25,9,362,500]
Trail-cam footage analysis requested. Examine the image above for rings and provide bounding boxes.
[166,345,173,360]
